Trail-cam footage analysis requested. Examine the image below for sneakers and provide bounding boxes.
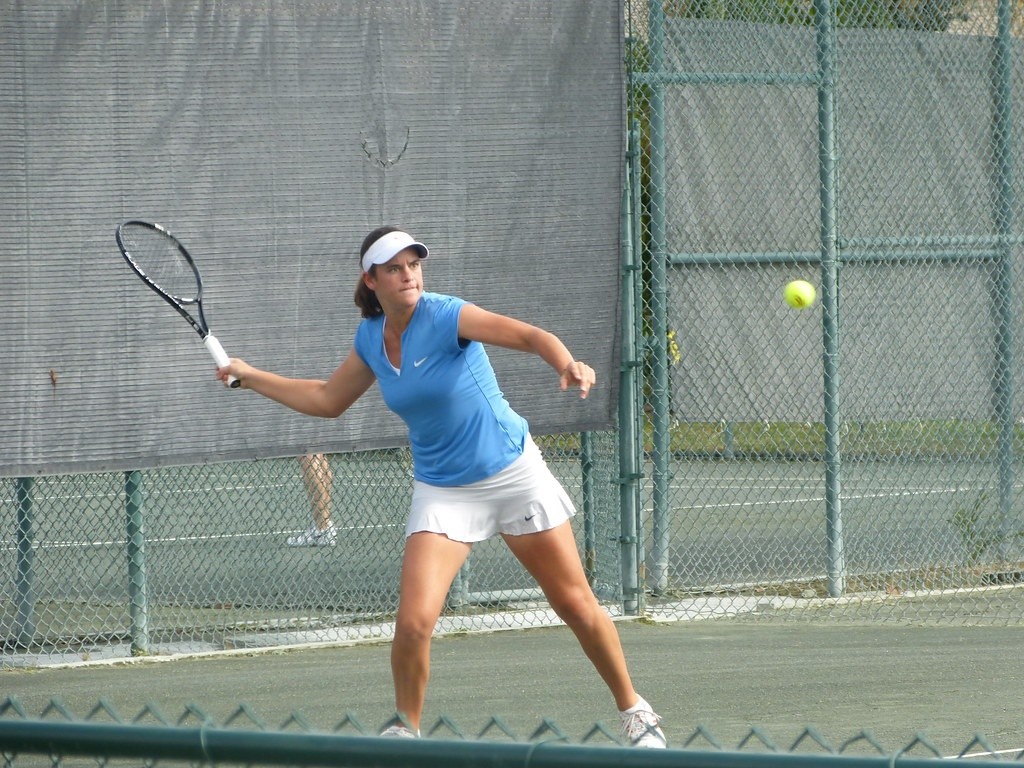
[618,693,667,750]
[287,520,339,547]
[381,723,421,740]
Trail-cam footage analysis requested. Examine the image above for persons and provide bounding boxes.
[212,225,672,750]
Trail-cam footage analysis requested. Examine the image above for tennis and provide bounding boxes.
[783,280,817,310]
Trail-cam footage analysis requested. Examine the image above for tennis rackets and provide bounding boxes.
[115,218,242,389]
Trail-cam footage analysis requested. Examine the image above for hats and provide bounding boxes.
[350,232,428,272]
